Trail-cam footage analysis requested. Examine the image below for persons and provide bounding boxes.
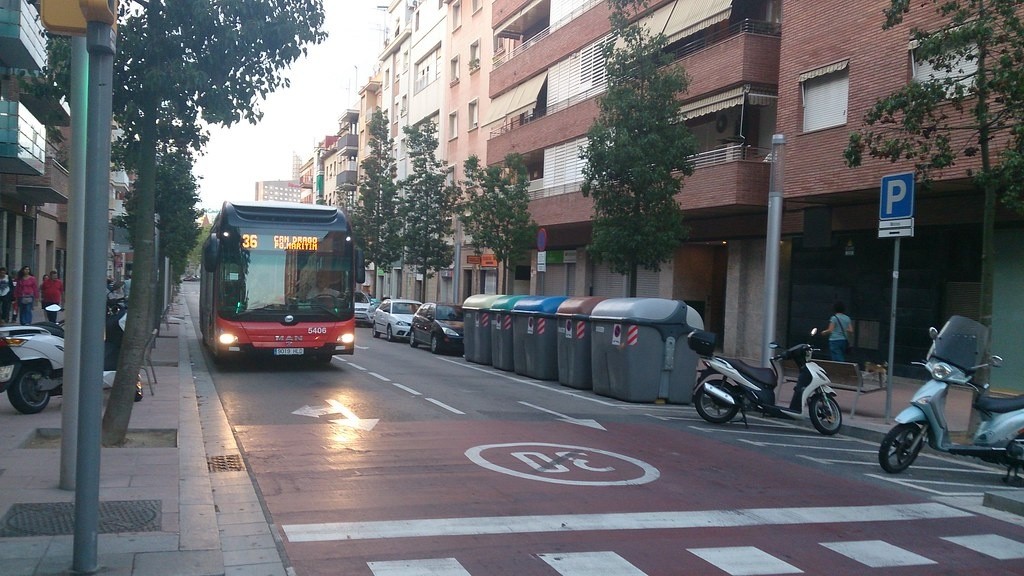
[0,266,132,326]
[305,274,341,301]
[818,300,854,384]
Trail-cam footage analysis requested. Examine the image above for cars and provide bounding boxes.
[353,291,376,328]
[409,302,465,354]
[372,299,423,342]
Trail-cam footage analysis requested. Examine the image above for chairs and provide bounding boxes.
[162,305,171,330]
[139,327,158,397]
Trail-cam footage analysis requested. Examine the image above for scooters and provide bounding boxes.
[878,314,1024,484]
[688,327,843,436]
[0,281,143,414]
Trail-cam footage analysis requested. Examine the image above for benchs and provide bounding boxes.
[776,358,888,419]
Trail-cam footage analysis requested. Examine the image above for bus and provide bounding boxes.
[200,200,366,365]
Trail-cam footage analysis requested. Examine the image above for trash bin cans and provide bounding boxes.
[555,296,609,390]
[511,296,570,380]
[461,293,506,364]
[589,297,704,404]
[489,294,530,372]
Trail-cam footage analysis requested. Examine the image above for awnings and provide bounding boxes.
[606,0,733,70]
[480,69,548,133]
